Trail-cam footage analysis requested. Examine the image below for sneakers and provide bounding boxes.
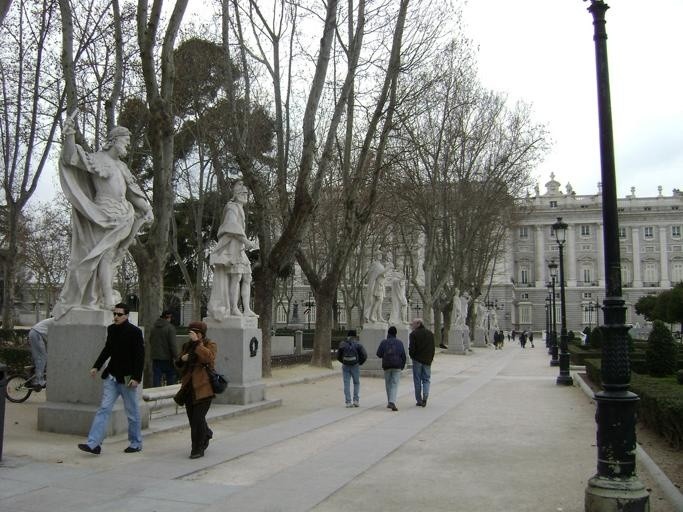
[78,444,100,454]
[416,400,426,406]
[346,399,359,408]
[387,403,397,411]
[125,447,141,452]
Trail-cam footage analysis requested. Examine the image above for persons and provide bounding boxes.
[451,288,498,330]
[394,268,408,324]
[52,117,155,320]
[174,321,217,459]
[494,327,591,351]
[376,326,406,411]
[206,181,260,322]
[78,304,144,455]
[29,317,54,388]
[337,330,367,407]
[409,318,435,407]
[150,311,179,387]
[364,250,390,322]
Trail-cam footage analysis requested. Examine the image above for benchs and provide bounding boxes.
[139,379,193,419]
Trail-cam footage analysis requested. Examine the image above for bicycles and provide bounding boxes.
[4,366,46,402]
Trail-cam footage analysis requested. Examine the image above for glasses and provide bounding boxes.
[114,312,126,317]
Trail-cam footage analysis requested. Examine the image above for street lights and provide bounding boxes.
[485,295,495,331]
[594,297,601,328]
[551,214,574,386]
[124,274,130,291]
[543,260,558,365]
[414,302,421,317]
[584,302,595,329]
[305,301,314,329]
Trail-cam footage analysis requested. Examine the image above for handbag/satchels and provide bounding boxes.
[206,367,227,393]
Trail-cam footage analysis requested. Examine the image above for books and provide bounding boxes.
[124,375,137,387]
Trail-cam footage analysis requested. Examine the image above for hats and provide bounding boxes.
[190,322,207,329]
[348,330,356,336]
[160,311,171,318]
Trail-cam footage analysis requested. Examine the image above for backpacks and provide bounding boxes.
[343,340,358,364]
